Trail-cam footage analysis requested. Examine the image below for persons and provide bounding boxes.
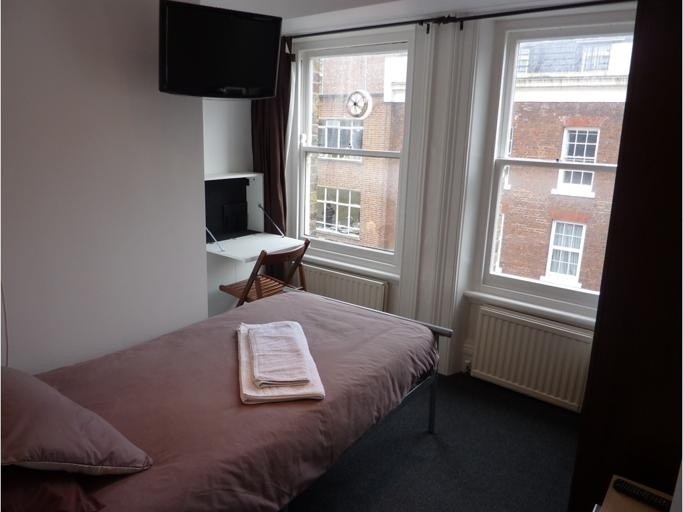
[322,203,334,227]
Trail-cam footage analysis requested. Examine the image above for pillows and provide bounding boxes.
[1,366,154,475]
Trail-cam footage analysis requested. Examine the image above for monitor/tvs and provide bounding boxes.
[158,0,282,101]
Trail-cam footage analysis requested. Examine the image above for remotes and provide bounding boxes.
[613,478,671,512]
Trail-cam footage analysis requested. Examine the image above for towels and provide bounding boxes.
[236,320,326,405]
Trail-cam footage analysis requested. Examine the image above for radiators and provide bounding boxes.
[470,305,594,414]
[293,261,389,313]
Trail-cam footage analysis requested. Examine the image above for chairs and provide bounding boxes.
[219,238,311,309]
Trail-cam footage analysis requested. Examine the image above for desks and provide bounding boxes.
[204,172,306,263]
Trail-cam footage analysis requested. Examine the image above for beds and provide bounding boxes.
[1,287,453,511]
